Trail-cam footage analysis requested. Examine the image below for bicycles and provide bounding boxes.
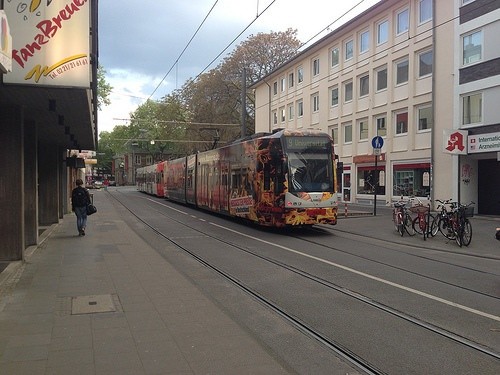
[392,194,476,247]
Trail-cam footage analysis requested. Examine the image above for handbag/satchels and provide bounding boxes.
[86,204,96,215]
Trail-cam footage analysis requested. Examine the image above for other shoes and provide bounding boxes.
[79,228,85,237]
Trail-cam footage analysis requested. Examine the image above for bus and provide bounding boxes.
[136,128,343,230]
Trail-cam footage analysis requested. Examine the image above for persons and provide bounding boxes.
[71,179,91,236]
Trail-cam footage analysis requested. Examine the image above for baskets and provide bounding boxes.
[461,206,473,217]
